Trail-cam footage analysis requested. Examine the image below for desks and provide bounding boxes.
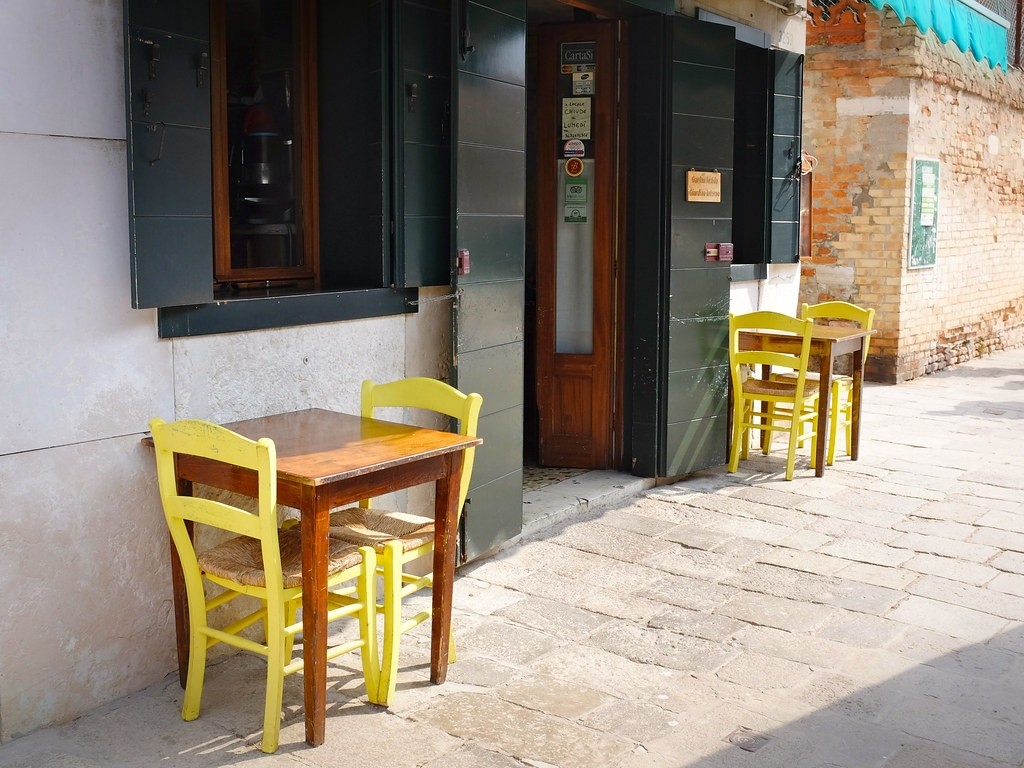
[140,406,484,748]
[728,323,877,477]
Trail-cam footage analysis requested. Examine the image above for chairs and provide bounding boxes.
[146,417,381,756]
[726,310,822,480]
[283,377,484,709]
[756,300,876,467]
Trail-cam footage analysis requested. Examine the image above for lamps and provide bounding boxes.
[240,46,283,138]
